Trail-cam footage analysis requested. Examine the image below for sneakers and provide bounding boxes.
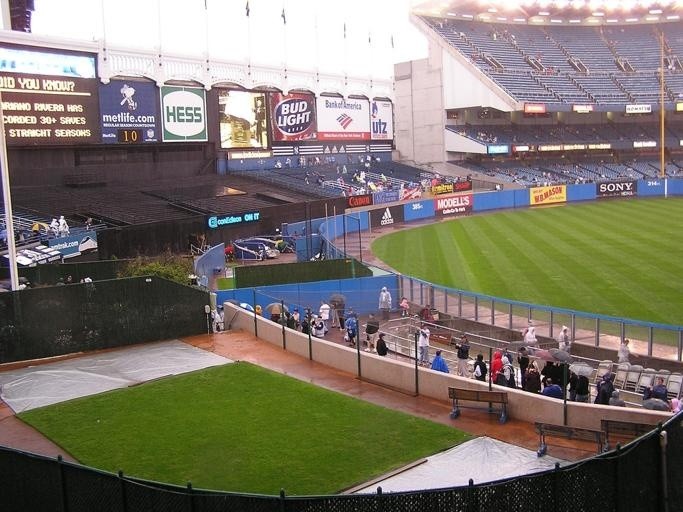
[364,348,370,352]
[372,349,377,352]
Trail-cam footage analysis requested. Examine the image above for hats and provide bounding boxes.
[379,334,385,337]
[460,335,465,338]
[436,350,441,355]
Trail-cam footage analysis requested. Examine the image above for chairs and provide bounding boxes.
[593,359,682,401]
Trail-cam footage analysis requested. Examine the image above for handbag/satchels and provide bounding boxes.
[344,335,350,342]
[496,373,514,387]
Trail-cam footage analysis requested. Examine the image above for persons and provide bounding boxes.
[647,377,668,402]
[253,299,389,357]
[269,152,437,199]
[400,296,410,317]
[440,159,683,186]
[211,305,225,333]
[378,287,393,323]
[460,125,599,145]
[0,214,96,291]
[249,99,265,145]
[617,339,630,363]
[418,324,625,408]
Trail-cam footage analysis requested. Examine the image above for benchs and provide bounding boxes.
[534,422,609,456]
[601,418,659,437]
[448,387,509,424]
